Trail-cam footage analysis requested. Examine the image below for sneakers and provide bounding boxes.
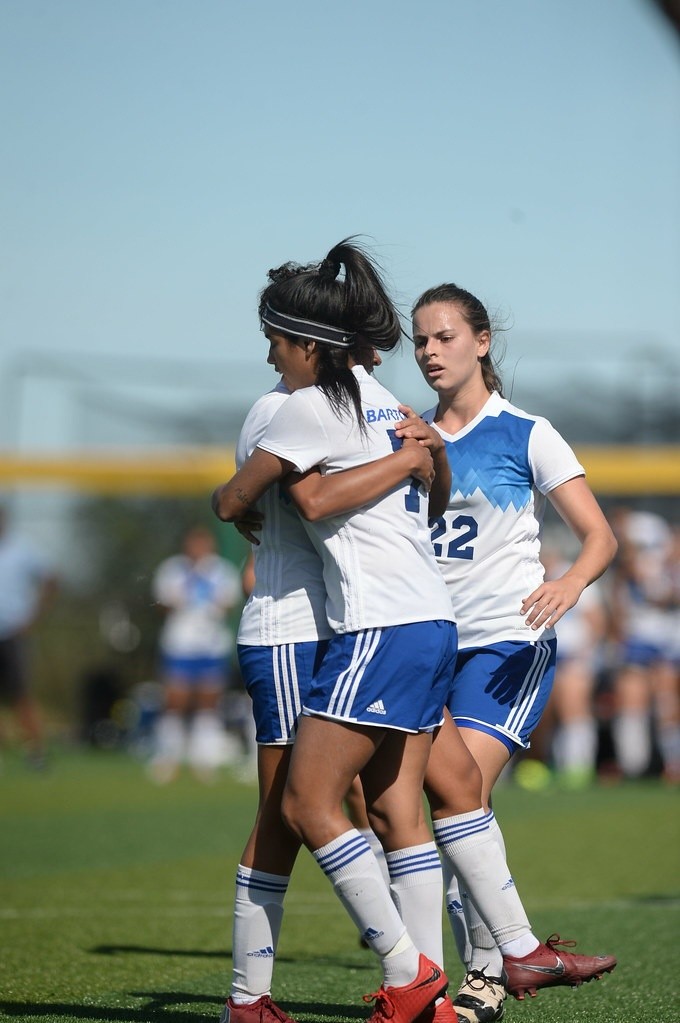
[413,995,458,1023]
[502,933,617,1000]
[217,996,297,1023]
[362,953,449,1023]
[450,964,507,1023]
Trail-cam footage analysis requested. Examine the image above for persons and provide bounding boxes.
[224,317,622,1023]
[209,231,462,1023]
[3,498,680,785]
[407,281,619,1023]
